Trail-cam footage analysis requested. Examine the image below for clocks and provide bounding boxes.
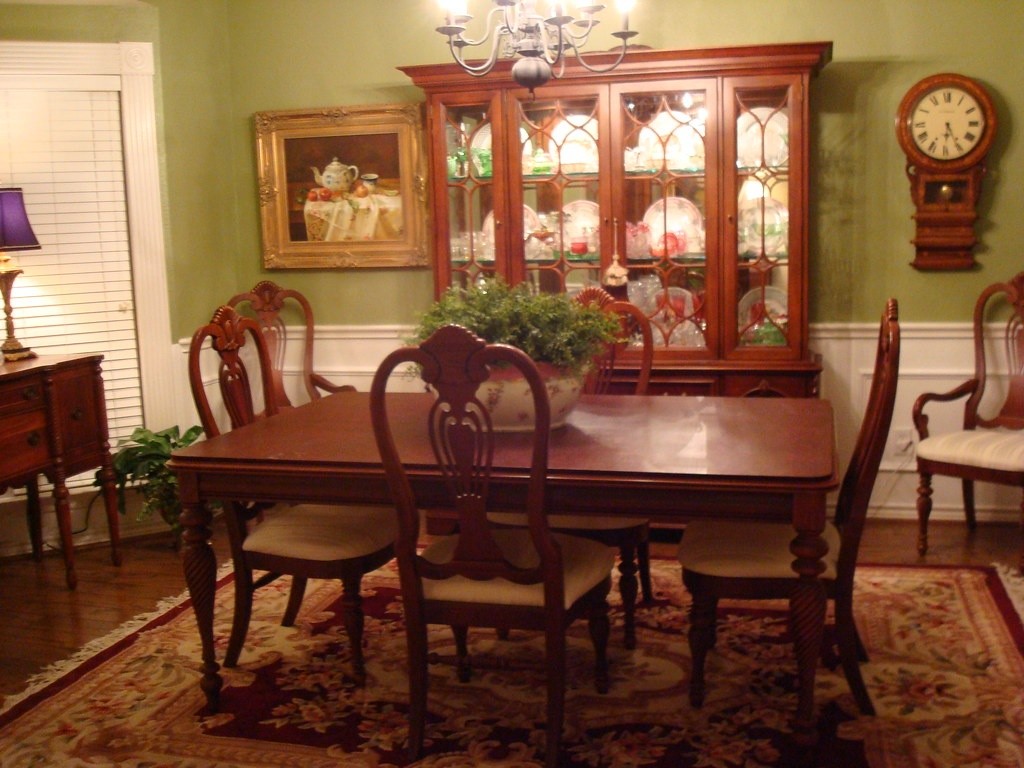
[894,73,996,272]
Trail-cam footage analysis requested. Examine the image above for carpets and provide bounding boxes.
[0,550,1024,768]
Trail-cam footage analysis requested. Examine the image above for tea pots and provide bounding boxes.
[526,148,556,174]
[310,156,358,194]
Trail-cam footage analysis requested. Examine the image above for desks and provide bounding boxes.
[0,353,122,590]
[167,392,837,743]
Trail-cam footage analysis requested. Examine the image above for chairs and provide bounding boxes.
[494,287,654,646]
[368,322,611,754]
[679,298,900,717]
[912,271,1024,557]
[226,280,350,419]
[189,307,406,687]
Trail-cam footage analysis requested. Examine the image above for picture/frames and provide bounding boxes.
[254,105,431,271]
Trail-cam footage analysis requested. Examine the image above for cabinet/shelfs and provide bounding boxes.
[396,40,836,523]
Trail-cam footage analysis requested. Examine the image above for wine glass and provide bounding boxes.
[643,291,706,347]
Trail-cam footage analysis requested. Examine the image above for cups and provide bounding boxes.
[453,147,491,178]
[449,227,650,259]
[361,173,378,193]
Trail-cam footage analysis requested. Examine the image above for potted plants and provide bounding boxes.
[93,425,225,550]
[399,271,623,432]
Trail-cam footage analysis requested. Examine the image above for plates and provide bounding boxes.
[548,114,599,168]
[738,287,788,338]
[737,197,788,254]
[737,106,789,167]
[482,202,541,260]
[470,121,532,176]
[645,287,695,333]
[549,199,599,252]
[637,110,700,169]
[642,196,702,251]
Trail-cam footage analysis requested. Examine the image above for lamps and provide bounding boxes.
[0,187,41,359]
[434,0,641,105]
